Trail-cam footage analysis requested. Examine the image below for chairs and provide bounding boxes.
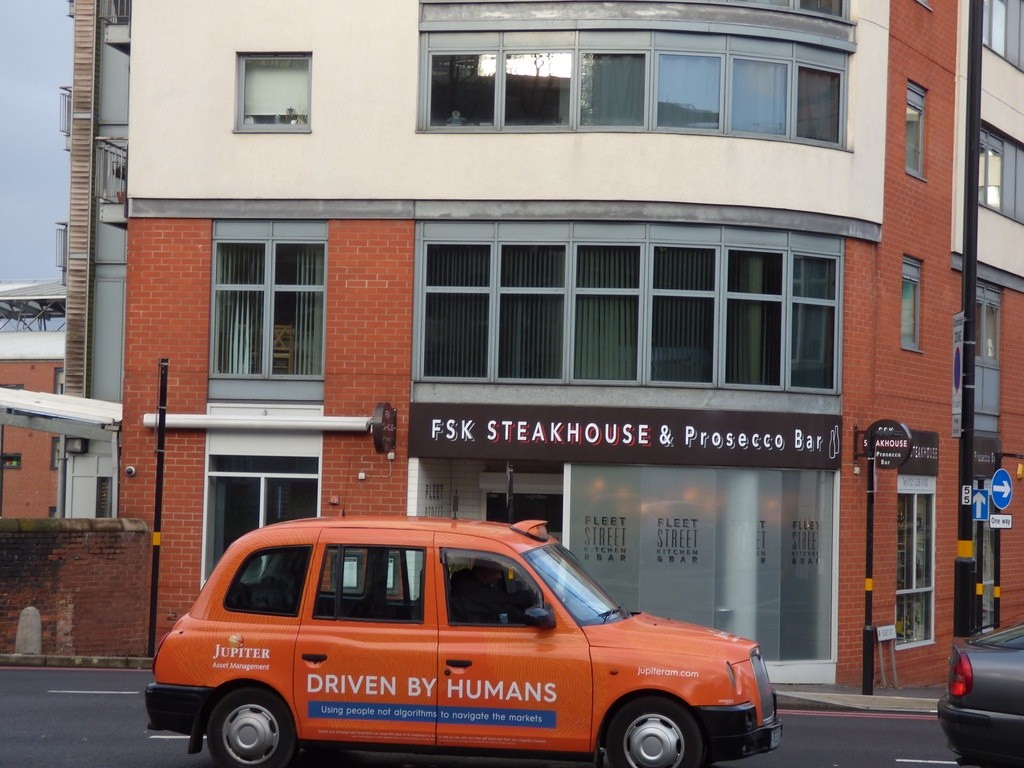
[230,576,302,614]
[449,568,477,625]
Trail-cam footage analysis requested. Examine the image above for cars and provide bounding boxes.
[146,514,784,768]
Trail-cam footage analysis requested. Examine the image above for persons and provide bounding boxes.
[455,558,540,625]
